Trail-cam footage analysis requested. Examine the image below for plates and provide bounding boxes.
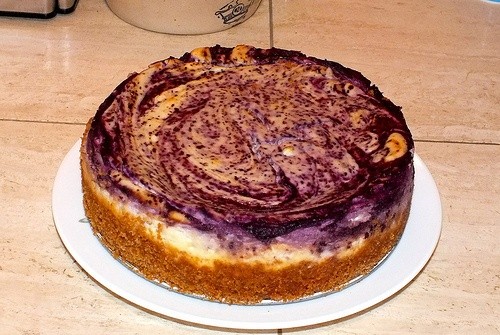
[52,117,443,331]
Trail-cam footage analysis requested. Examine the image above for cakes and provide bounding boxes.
[80,43,416,304]
